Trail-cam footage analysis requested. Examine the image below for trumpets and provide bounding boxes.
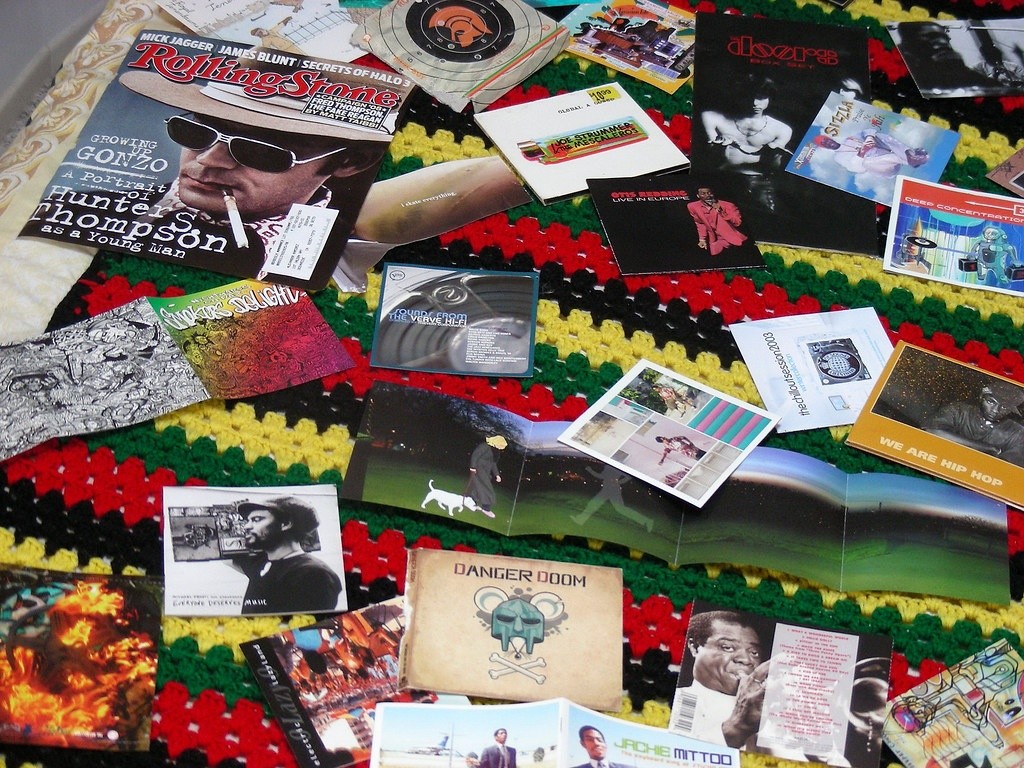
[735,657,891,732]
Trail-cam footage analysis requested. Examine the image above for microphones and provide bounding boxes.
[705,195,722,212]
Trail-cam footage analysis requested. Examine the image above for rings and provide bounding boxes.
[748,675,761,683]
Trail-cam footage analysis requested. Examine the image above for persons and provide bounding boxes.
[974,43,1024,88]
[470,728,517,768]
[652,385,697,418]
[119,45,387,281]
[465,751,478,768]
[920,381,1024,467]
[687,183,760,269]
[813,129,929,177]
[331,156,531,296]
[238,497,342,615]
[656,436,706,465]
[702,75,793,211]
[670,612,851,768]
[897,21,1005,90]
[570,725,638,768]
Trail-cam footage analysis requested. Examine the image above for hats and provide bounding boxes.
[119,47,414,141]
[237,496,320,534]
[815,135,828,148]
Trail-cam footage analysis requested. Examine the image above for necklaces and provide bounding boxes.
[734,113,768,136]
[834,143,862,152]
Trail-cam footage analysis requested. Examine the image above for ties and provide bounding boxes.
[598,761,604,768]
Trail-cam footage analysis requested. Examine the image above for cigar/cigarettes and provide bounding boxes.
[219,186,249,249]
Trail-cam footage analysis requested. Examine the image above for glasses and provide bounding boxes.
[164,112,350,173]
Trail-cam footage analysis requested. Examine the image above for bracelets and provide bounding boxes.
[997,449,1001,455]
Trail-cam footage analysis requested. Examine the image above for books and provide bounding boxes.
[690,12,880,258]
[586,172,768,277]
[15,28,414,290]
